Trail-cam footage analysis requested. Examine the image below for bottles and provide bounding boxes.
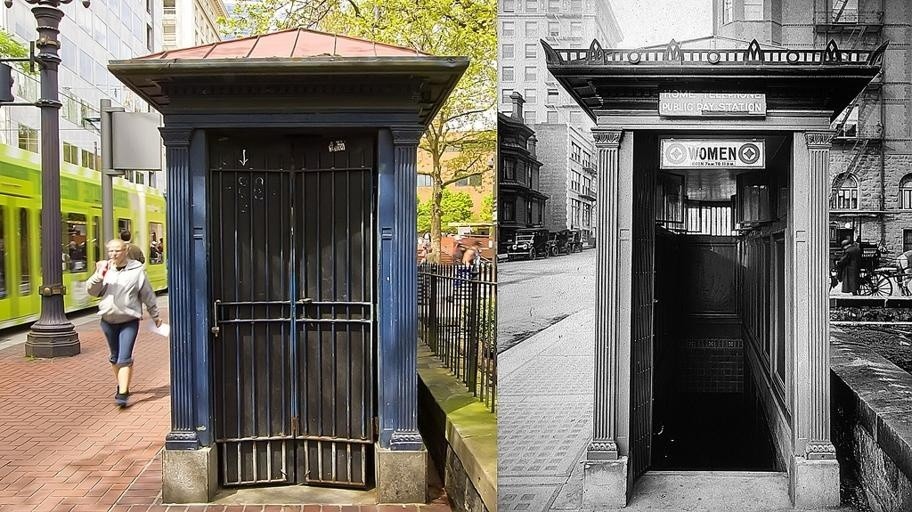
[98,259,115,280]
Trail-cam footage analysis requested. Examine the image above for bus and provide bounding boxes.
[0,141,168,333]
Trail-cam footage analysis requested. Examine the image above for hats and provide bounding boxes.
[841,240,851,249]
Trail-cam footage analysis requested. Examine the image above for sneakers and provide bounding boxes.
[115,385,130,408]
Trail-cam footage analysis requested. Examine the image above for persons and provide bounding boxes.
[463,242,482,272]
[426,249,437,264]
[836,240,861,296]
[418,246,428,265]
[64,231,163,405]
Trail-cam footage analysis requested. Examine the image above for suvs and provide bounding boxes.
[506,227,583,261]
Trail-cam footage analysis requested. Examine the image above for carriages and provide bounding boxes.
[831,238,912,297]
